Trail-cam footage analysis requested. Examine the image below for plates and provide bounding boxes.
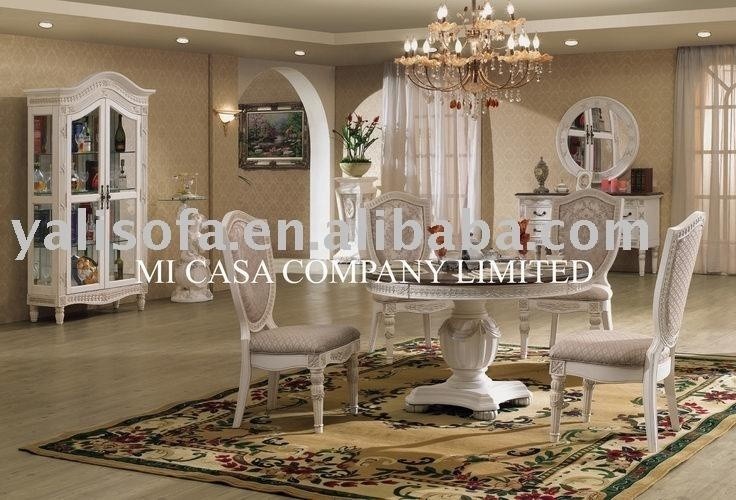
[412,247,584,270]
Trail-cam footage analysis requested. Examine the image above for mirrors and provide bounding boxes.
[555,95,640,184]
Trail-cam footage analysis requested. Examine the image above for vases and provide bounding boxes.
[339,162,371,178]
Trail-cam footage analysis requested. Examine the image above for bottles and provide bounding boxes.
[30,113,126,286]
[554,179,570,193]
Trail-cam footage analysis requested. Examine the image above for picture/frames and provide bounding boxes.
[238,102,310,169]
[576,170,594,191]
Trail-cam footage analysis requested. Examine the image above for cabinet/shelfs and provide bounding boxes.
[515,192,664,277]
[23,71,156,324]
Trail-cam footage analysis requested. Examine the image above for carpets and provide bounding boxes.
[19,336,736,500]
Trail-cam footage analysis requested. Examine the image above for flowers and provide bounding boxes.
[332,112,380,163]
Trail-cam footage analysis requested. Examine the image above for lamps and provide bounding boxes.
[533,157,549,194]
[214,109,242,137]
[393,0,554,122]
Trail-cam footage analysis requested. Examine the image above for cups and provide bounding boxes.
[599,176,628,194]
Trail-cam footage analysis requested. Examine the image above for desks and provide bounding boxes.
[333,177,378,264]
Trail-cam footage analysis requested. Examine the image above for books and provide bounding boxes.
[631,167,654,193]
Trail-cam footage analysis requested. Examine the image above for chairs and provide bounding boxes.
[517,188,625,358]
[549,210,706,452]
[217,210,361,434]
[363,190,455,365]
